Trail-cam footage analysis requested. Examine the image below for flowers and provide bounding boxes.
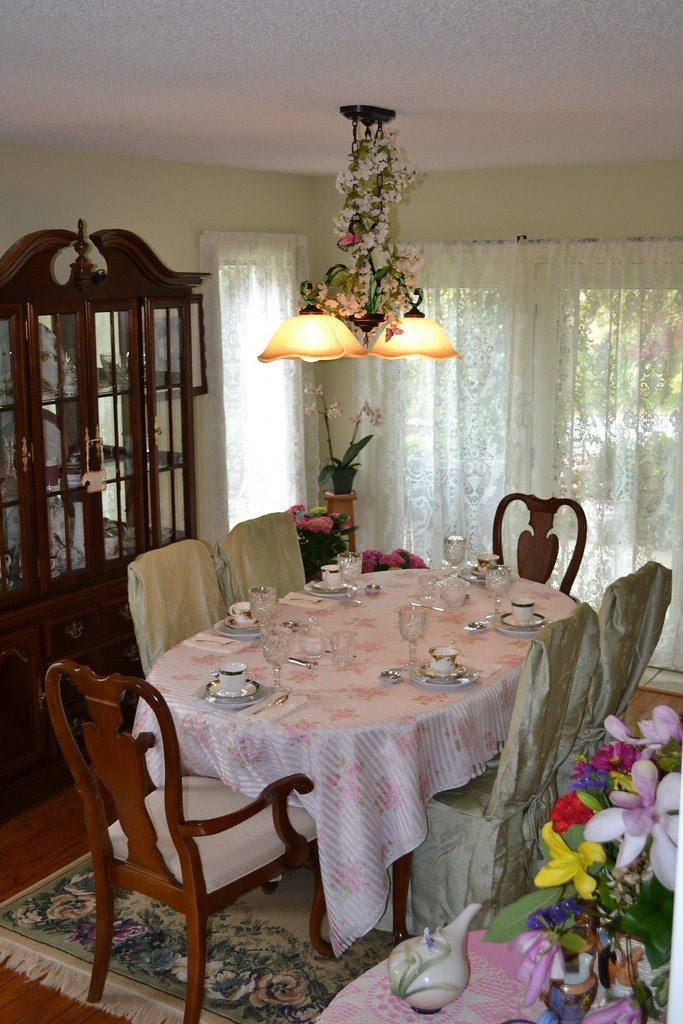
[485,703,683,1023]
[361,547,426,574]
[287,503,356,584]
[303,383,382,485]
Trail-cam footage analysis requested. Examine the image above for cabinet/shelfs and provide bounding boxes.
[0,218,209,820]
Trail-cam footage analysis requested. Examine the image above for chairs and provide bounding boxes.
[213,513,305,612]
[126,539,229,677]
[556,560,674,795]
[378,603,599,932]
[491,493,588,598]
[45,660,332,1024]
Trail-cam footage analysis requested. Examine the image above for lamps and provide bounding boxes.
[258,103,460,359]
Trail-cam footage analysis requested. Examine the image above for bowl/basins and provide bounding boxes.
[101,355,146,389]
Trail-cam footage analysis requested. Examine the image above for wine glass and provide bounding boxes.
[398,606,425,672]
[259,626,292,694]
[337,552,363,608]
[443,535,466,577]
[248,586,277,648]
[484,565,514,621]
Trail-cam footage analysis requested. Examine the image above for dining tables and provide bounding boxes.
[130,568,577,956]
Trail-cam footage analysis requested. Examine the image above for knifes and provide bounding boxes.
[410,603,444,612]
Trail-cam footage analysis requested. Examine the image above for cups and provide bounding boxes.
[228,601,256,625]
[44,459,59,491]
[511,598,536,624]
[419,552,500,608]
[66,459,82,486]
[429,640,459,675]
[320,564,342,587]
[331,631,357,669]
[218,662,254,695]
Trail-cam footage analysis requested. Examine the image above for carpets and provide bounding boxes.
[0,852,406,1024]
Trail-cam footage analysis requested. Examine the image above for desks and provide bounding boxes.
[316,930,547,1024]
[326,494,356,552]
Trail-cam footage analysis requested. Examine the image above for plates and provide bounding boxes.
[213,615,260,639]
[280,621,301,632]
[8,497,83,568]
[463,612,552,636]
[311,580,348,590]
[380,670,403,683]
[206,680,260,700]
[37,323,67,392]
[364,584,381,593]
[199,684,270,709]
[406,664,479,690]
[457,566,486,584]
[304,580,359,600]
[421,662,466,680]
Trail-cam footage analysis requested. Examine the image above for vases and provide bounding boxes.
[330,468,353,496]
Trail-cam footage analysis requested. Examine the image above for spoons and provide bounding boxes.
[290,598,326,604]
[253,694,288,714]
